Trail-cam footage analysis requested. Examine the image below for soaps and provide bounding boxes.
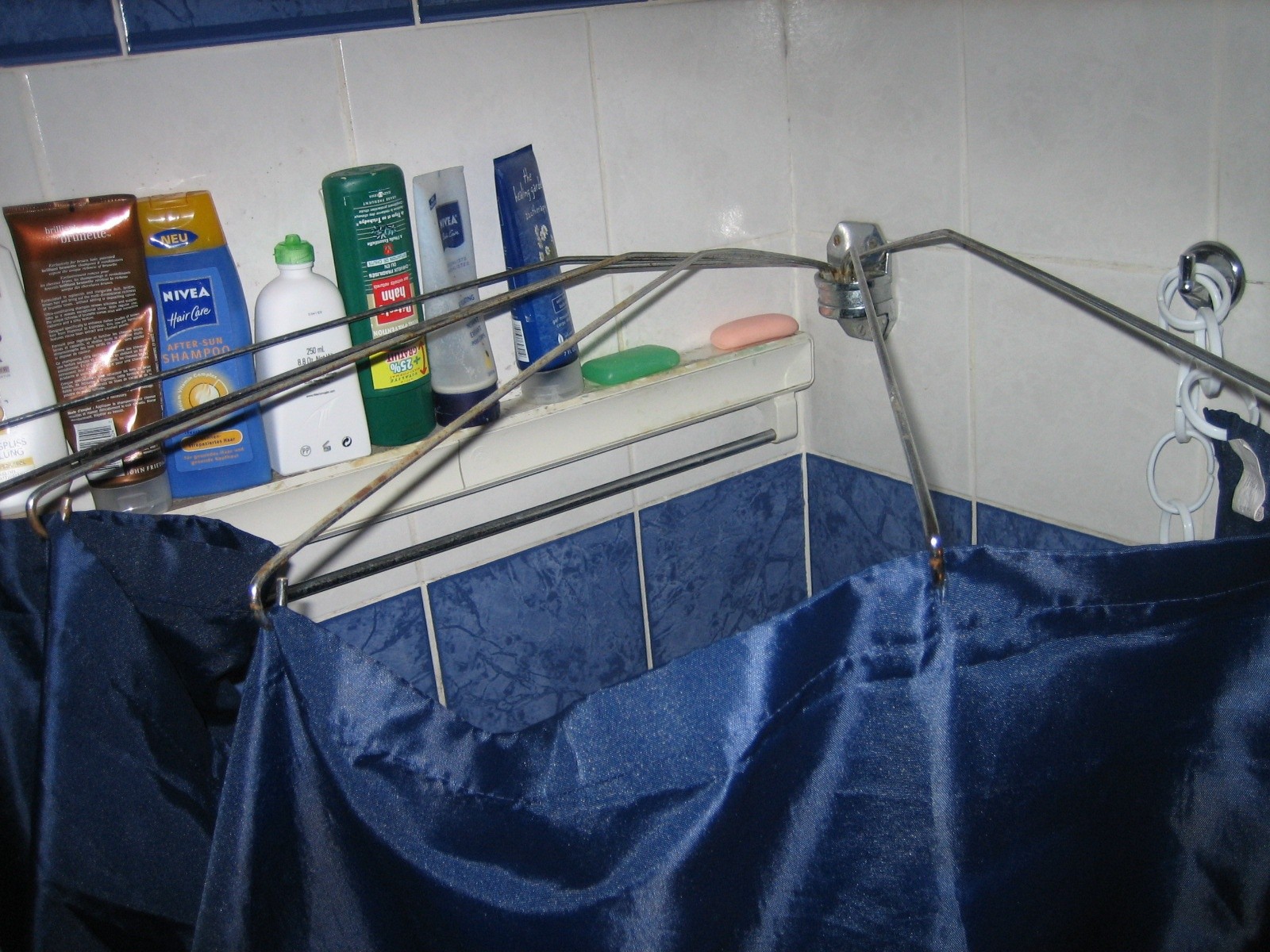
[580,343,680,384]
[708,311,798,352]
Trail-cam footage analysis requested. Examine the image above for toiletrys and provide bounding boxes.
[493,142,584,405]
[411,165,502,426]
[255,232,372,478]
[0,247,71,523]
[136,190,227,259]
[147,245,274,500]
[321,164,436,449]
[1,194,172,517]
